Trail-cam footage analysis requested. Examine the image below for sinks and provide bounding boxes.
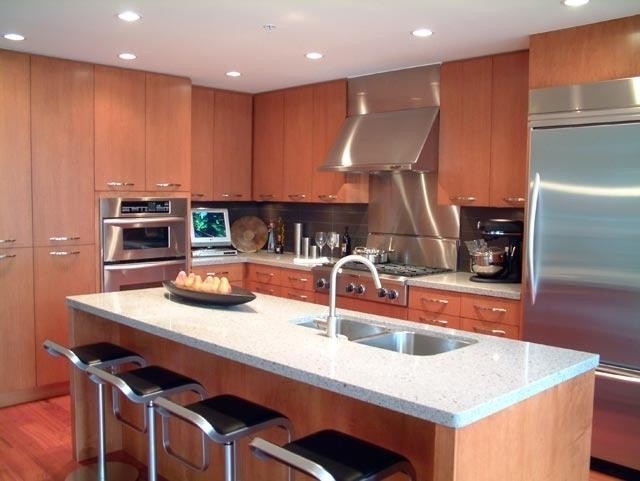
[309,318,387,339]
[358,331,471,355]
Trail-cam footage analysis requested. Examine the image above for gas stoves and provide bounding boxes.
[323,260,452,277]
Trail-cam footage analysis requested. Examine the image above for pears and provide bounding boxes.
[175,270,231,295]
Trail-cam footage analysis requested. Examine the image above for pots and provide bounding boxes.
[353,247,389,265]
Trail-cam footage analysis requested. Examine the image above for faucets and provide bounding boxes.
[312,254,382,338]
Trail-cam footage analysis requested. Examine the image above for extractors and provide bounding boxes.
[316,64,440,176]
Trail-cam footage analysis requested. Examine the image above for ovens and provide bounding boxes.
[100,197,187,293]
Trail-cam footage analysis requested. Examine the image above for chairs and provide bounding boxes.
[249,427,419,480]
[39,336,143,481]
[86,366,211,480]
[156,394,294,480]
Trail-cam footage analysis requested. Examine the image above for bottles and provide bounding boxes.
[274,236,283,254]
[267,224,275,253]
[340,227,352,256]
[310,241,319,258]
[301,232,311,258]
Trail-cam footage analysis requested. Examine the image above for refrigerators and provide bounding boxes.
[521,77,639,474]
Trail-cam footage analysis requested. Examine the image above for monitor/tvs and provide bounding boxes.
[190,208,232,249]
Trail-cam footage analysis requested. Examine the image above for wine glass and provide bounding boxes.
[314,232,327,264]
[326,232,339,262]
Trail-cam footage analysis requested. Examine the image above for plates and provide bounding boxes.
[162,277,257,304]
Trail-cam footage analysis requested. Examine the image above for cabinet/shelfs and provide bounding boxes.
[32,51,96,403]
[0,50,37,411]
[251,77,346,204]
[192,262,244,291]
[192,85,252,203]
[409,286,521,339]
[438,48,529,208]
[244,262,314,305]
[95,63,193,191]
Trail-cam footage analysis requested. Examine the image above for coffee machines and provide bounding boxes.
[470,218,522,284]
